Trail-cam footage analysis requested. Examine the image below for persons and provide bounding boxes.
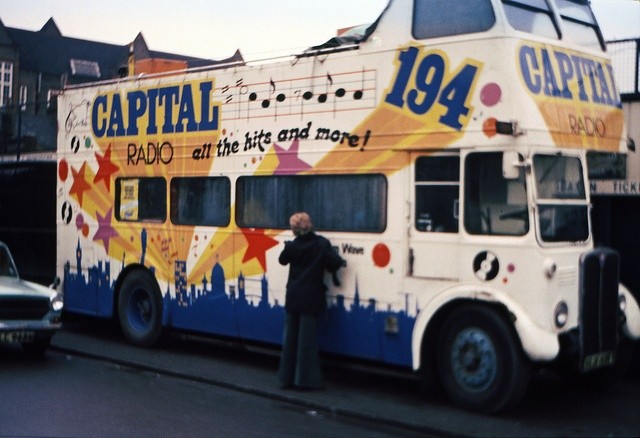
[278,213,343,393]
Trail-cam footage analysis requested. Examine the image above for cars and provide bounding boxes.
[0,240,64,363]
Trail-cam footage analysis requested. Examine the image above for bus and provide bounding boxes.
[46,2,639,416]
